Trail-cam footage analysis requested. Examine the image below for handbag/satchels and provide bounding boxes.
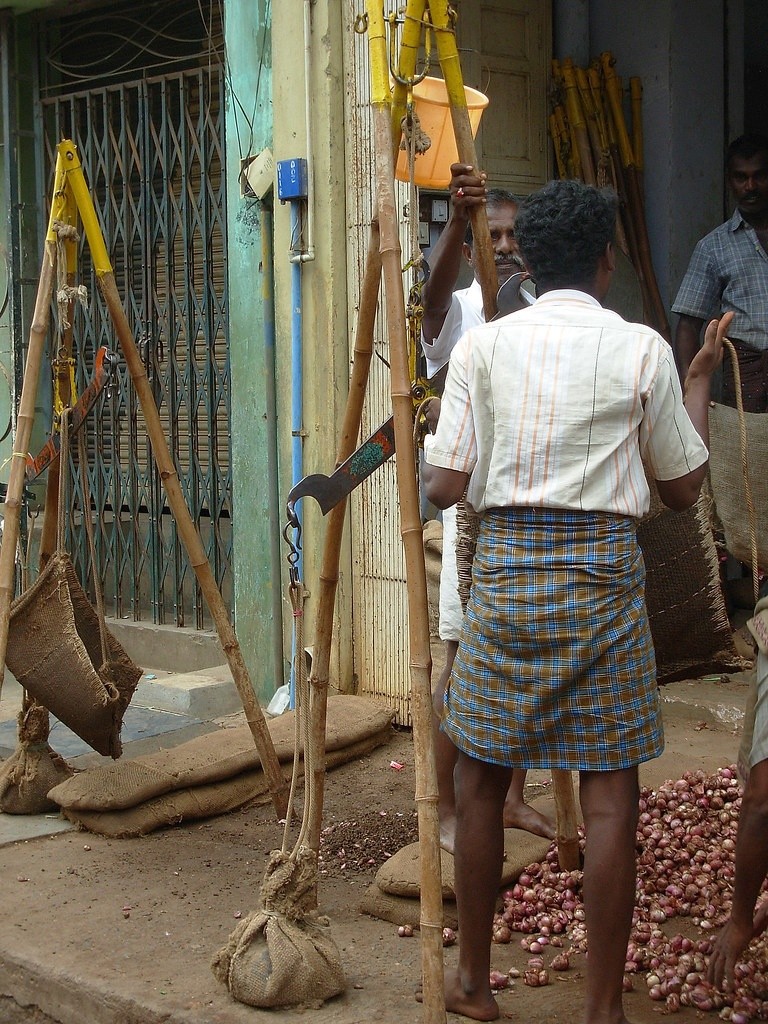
[6,405,144,758]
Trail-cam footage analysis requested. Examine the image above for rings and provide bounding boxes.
[455,187,465,199]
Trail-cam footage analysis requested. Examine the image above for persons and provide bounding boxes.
[412,177,736,1024]
[706,579,768,993]
[666,134,768,413]
[419,162,558,863]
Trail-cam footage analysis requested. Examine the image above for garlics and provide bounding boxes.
[490,762,768,1024]
[443,928,457,948]
[397,923,415,940]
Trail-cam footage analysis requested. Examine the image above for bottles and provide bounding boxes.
[266,680,290,718]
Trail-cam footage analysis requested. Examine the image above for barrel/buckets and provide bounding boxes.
[387,48,490,190]
